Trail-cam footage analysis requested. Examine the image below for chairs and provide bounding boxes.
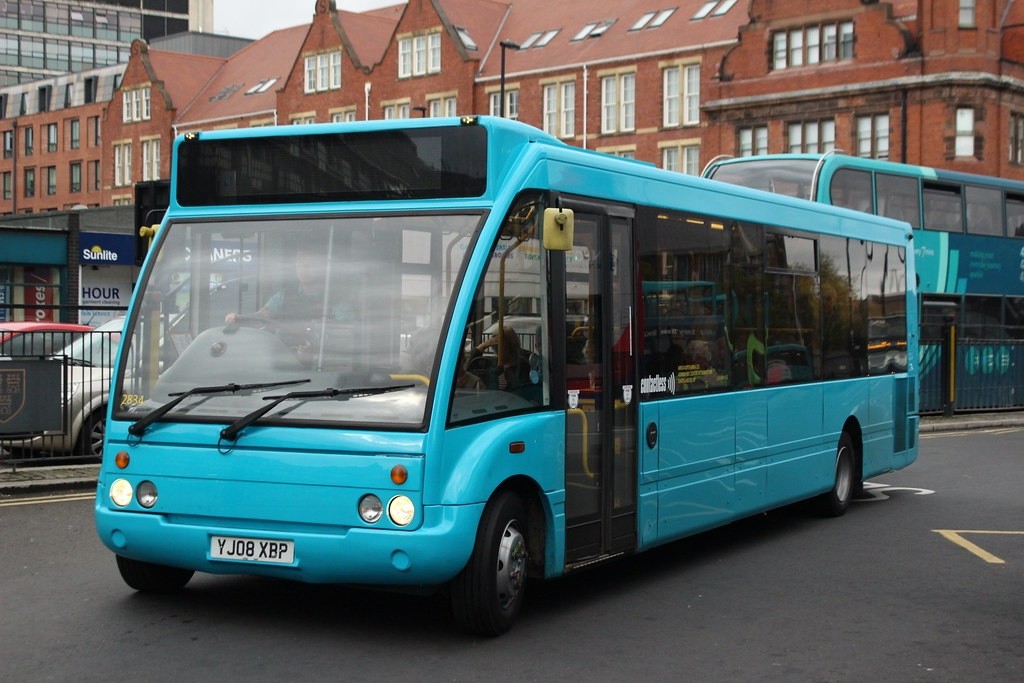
[766,343,814,381]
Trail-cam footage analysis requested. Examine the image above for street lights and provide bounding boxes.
[500,38,521,118]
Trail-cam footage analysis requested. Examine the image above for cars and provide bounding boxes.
[0,314,179,465]
[463,315,544,358]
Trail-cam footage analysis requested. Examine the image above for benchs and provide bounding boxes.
[466,357,498,389]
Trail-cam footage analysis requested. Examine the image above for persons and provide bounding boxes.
[463,324,530,392]
[662,281,855,391]
[223,250,356,362]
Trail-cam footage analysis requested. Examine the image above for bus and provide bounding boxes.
[94,114,922,642]
[698,149,1023,421]
